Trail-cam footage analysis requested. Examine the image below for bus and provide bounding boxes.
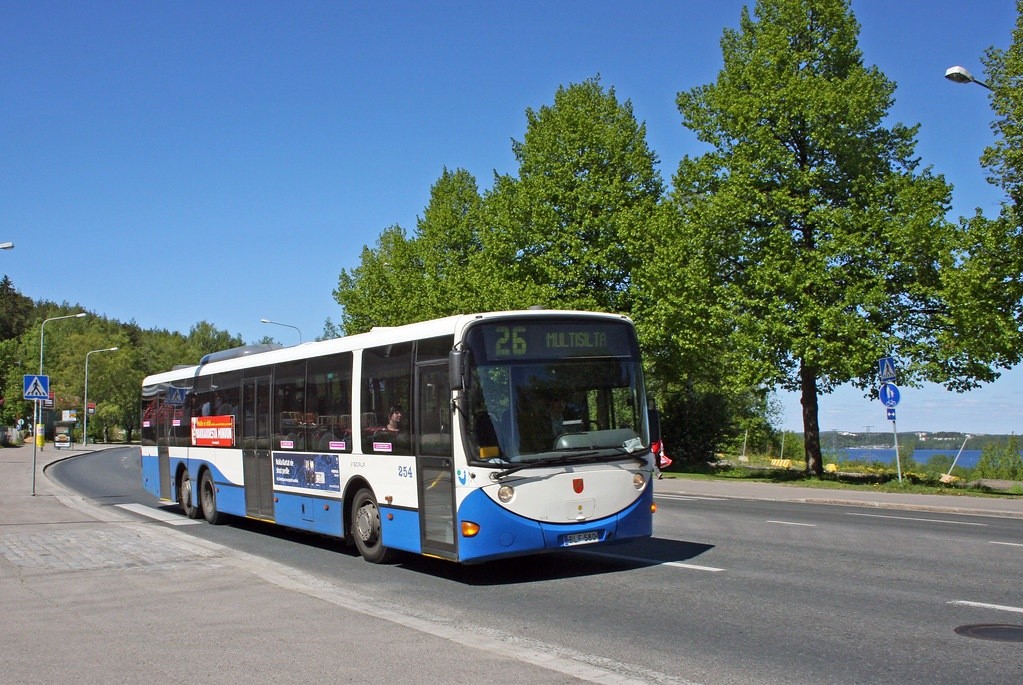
[136,308,654,567]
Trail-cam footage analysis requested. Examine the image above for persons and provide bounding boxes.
[646,398,665,480]
[189,382,589,457]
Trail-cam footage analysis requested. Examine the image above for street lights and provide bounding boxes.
[260,319,302,345]
[82,347,118,446]
[38,312,88,453]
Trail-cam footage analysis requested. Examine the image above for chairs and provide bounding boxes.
[142,406,582,472]
